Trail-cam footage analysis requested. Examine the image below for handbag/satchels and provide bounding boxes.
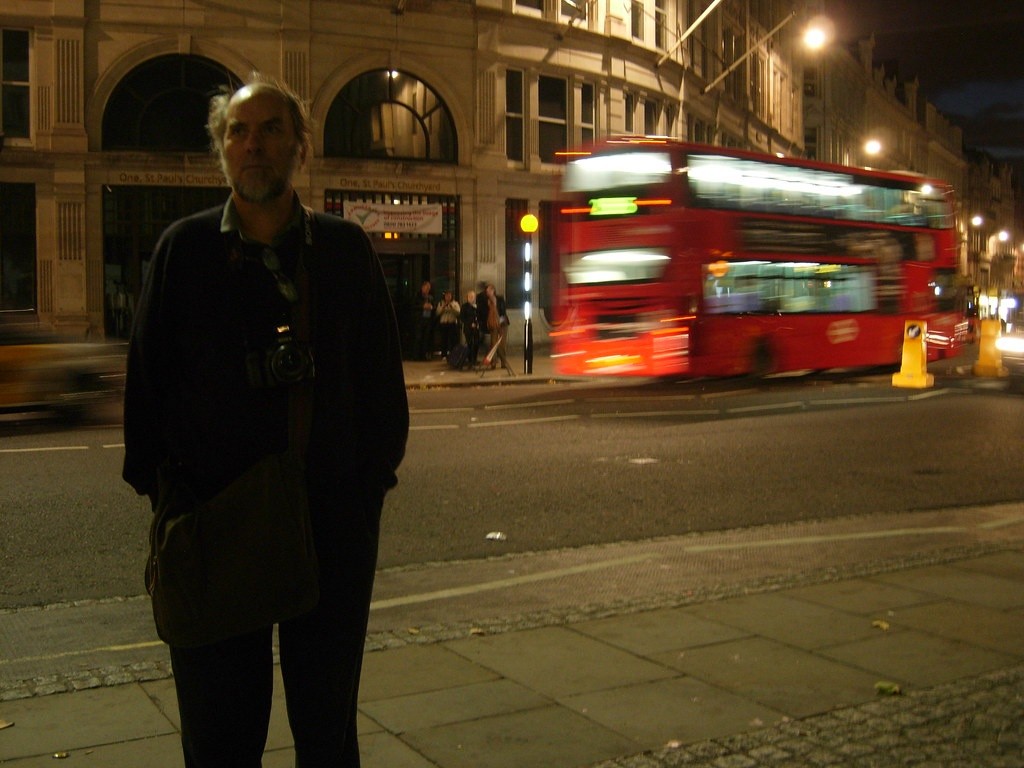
[147,458,320,644]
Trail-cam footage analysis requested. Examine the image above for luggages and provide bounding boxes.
[448,343,469,369]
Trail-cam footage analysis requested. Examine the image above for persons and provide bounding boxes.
[436,290,462,363]
[411,281,439,361]
[478,284,510,371]
[459,291,480,370]
[116,73,413,768]
[477,280,490,349]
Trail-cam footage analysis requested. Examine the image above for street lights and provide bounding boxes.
[519,213,538,376]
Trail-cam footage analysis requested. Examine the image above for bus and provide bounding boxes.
[545,133,980,386]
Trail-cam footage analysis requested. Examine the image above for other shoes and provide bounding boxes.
[490,365,506,369]
